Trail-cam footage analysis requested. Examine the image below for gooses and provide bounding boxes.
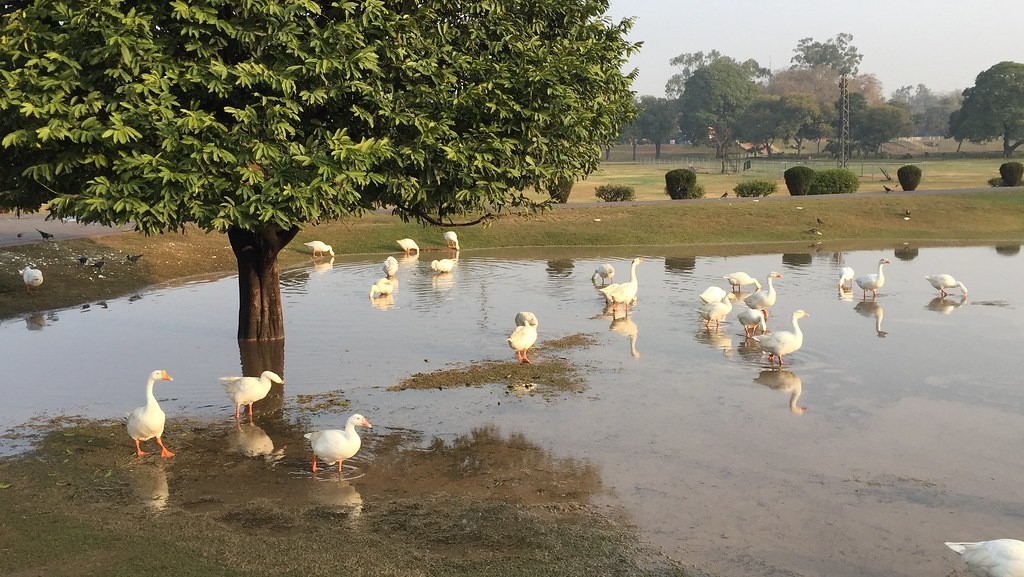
[126,369,176,458]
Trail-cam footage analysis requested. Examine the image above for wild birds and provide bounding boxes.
[91,256,105,270]
[720,190,728,199]
[882,185,894,194]
[906,209,911,215]
[17,233,22,239]
[126,254,143,264]
[817,219,824,225]
[809,228,818,233]
[36,229,53,241]
[78,255,89,267]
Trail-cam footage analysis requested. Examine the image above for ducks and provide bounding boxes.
[609,257,644,311]
[699,286,726,303]
[743,272,783,309]
[753,371,807,415]
[855,258,891,299]
[443,231,460,250]
[692,293,738,327]
[923,296,966,315]
[368,277,394,299]
[592,264,615,285]
[722,272,761,291]
[750,310,810,363]
[382,256,399,276]
[923,274,968,296]
[943,538,1024,575]
[609,319,641,359]
[303,240,335,257]
[395,238,420,253]
[431,258,454,273]
[505,319,538,364]
[18,265,44,296]
[599,283,638,306]
[216,371,284,418]
[837,267,854,290]
[515,311,538,329]
[854,302,888,338]
[303,414,372,472]
